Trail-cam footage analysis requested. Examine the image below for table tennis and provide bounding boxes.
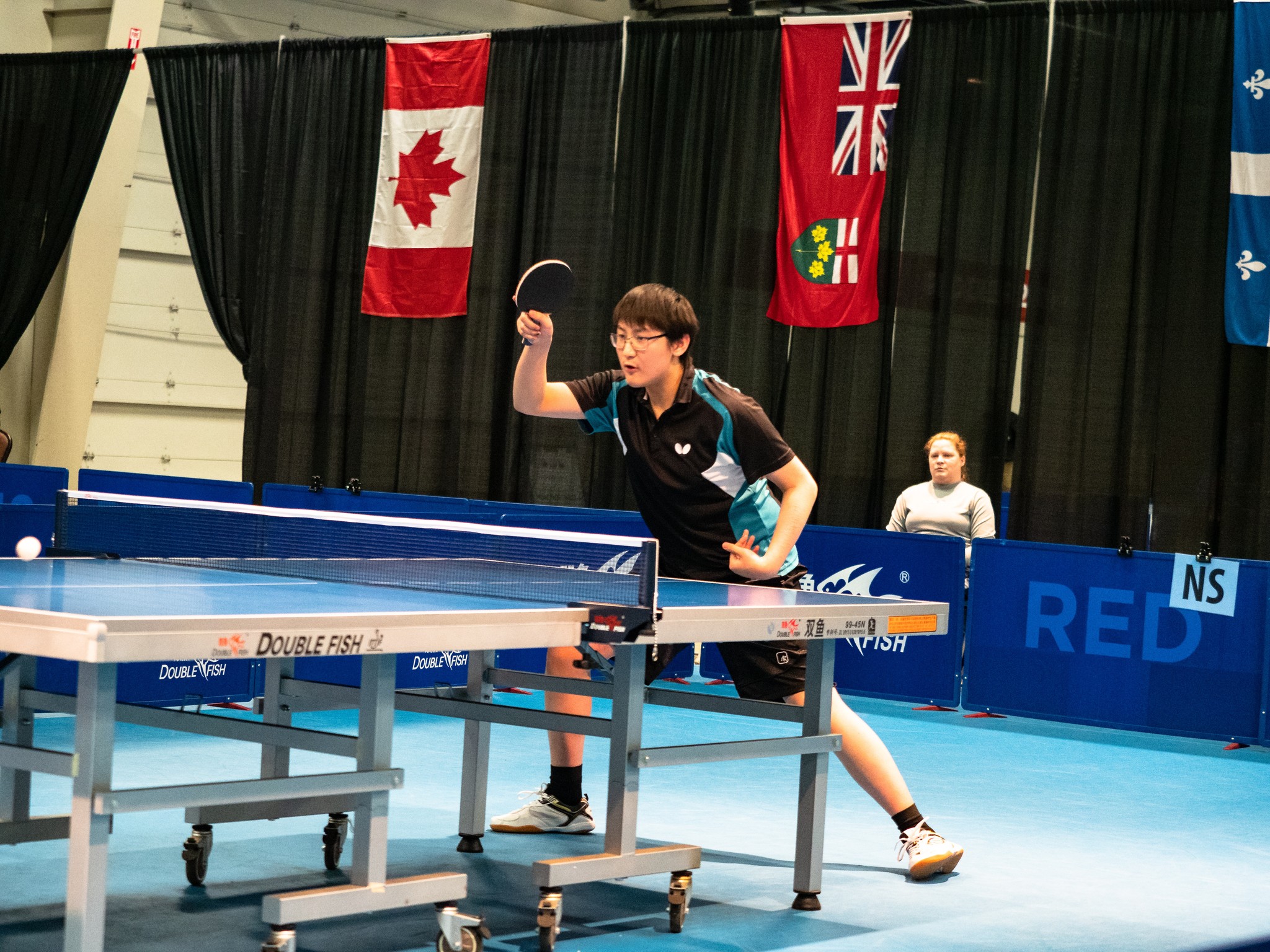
[14,536,42,562]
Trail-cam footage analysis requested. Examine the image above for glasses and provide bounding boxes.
[610,332,668,351]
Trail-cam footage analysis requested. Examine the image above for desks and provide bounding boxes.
[0,485,954,952]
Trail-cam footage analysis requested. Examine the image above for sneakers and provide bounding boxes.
[892,815,964,877]
[490,780,595,833]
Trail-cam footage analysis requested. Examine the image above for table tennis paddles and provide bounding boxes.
[515,259,574,346]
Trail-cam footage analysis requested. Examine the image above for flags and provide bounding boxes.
[765,11,912,327]
[360,33,491,318]
[1225,1,1270,348]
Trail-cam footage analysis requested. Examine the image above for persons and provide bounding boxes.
[885,431,997,601]
[490,282,964,879]
